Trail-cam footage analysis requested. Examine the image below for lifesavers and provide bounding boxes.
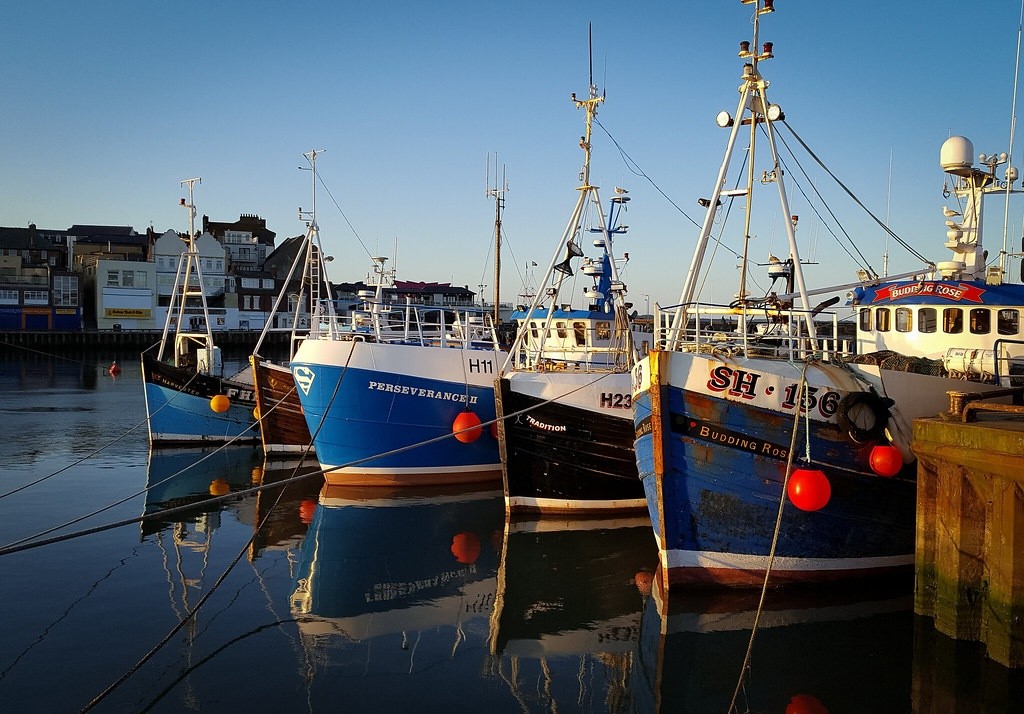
[836,391,889,442]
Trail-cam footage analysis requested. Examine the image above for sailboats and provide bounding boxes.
[134,1,1024,714]
